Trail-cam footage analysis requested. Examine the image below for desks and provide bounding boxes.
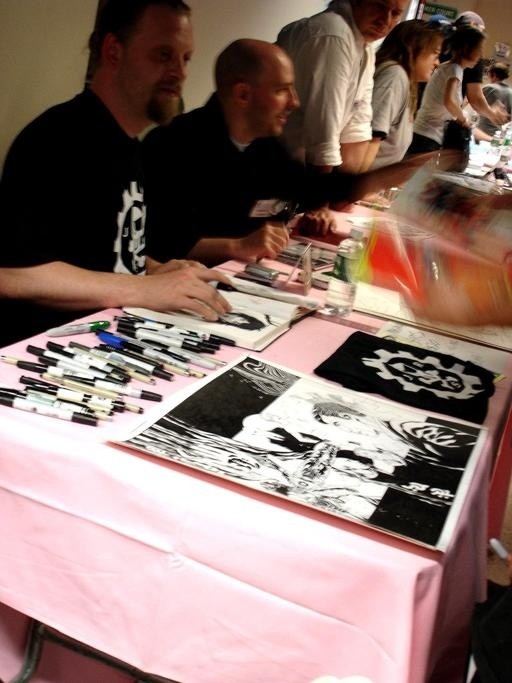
[0,121,512,683]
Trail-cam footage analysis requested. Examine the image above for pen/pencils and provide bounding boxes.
[208,280,233,291]
[0,313,235,426]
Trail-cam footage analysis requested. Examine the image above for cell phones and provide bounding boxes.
[244,263,279,281]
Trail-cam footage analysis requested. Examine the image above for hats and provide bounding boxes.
[426,9,485,31]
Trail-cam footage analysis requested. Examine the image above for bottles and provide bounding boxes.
[485,129,512,168]
[323,227,368,318]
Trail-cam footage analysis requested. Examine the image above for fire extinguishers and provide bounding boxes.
[416,0,426,21]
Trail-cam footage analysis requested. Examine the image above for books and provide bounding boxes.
[384,161,512,264]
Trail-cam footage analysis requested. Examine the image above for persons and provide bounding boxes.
[0,0,235,343]
[275,0,512,173]
[203,308,265,331]
[466,193,512,235]
[141,36,340,267]
[309,399,479,470]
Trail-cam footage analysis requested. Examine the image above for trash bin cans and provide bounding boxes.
[464,585,512,683]
